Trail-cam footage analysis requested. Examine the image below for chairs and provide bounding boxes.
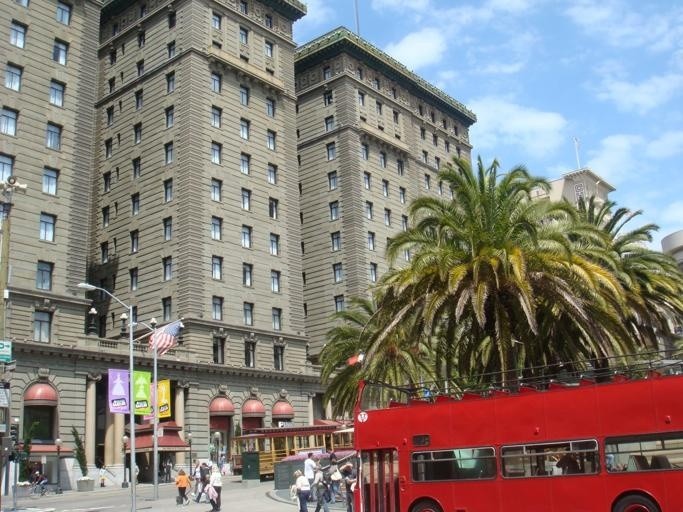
[627,455,671,472]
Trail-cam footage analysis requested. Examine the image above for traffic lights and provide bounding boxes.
[10,425,20,442]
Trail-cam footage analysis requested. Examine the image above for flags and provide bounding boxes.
[150,321,180,356]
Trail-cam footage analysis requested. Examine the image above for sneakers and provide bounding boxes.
[191,492,197,497]
[186,500,189,504]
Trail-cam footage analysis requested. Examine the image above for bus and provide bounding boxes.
[354,347,683,512]
[231,425,354,475]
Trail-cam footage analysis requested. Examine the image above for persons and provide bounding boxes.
[189,453,227,512]
[305,448,343,512]
[175,469,192,507]
[295,469,310,512]
[31,471,48,494]
[555,448,581,475]
[339,460,357,512]
[159,458,173,485]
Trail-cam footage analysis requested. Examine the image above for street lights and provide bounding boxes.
[78,282,185,512]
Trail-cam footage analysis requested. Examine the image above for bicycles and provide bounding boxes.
[29,482,50,499]
